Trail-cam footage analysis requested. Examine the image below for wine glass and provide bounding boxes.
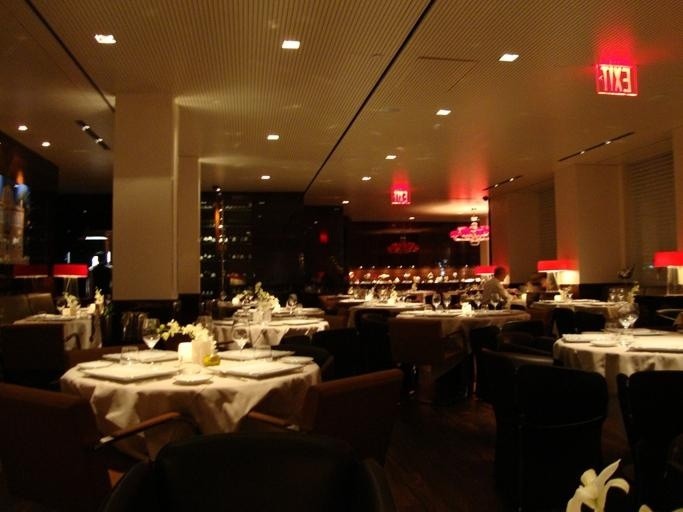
[286,295,296,318]
[56,299,65,312]
[616,303,640,333]
[432,293,500,312]
[117,318,272,368]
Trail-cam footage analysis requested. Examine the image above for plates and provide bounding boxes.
[539,300,555,303]
[590,302,615,306]
[77,349,313,384]
[562,324,683,351]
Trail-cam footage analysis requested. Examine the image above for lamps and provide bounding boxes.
[654,252,683,269]
[475,266,497,275]
[537,258,576,272]
[448,216,490,246]
[51,263,89,278]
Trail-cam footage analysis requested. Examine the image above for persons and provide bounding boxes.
[91,252,112,298]
[483,265,513,304]
